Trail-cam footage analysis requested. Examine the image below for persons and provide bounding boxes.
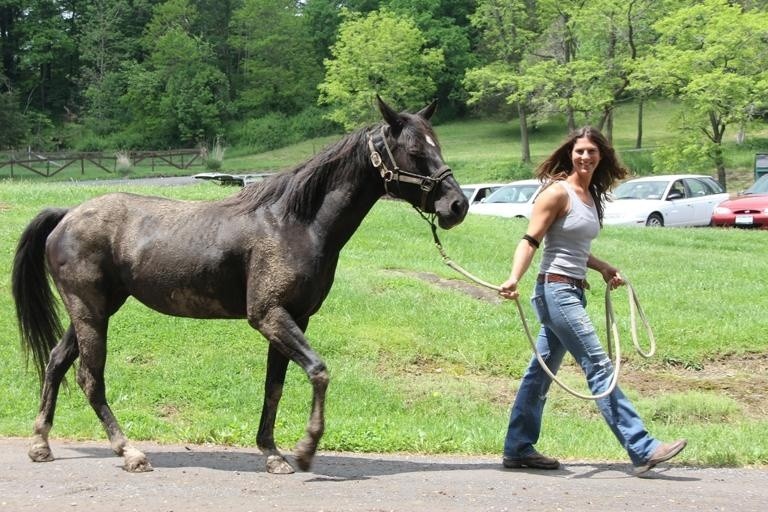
[499,125,688,476]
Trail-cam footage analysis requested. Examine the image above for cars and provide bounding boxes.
[467,179,547,222]
[191,172,271,187]
[600,174,737,229]
[711,173,767,229]
[459,182,508,211]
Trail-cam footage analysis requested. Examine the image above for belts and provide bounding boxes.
[538,274,591,290]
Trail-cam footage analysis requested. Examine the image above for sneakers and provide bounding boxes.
[633,442,686,474]
[503,451,559,469]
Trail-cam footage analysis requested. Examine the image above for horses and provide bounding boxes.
[10,92,470,476]
